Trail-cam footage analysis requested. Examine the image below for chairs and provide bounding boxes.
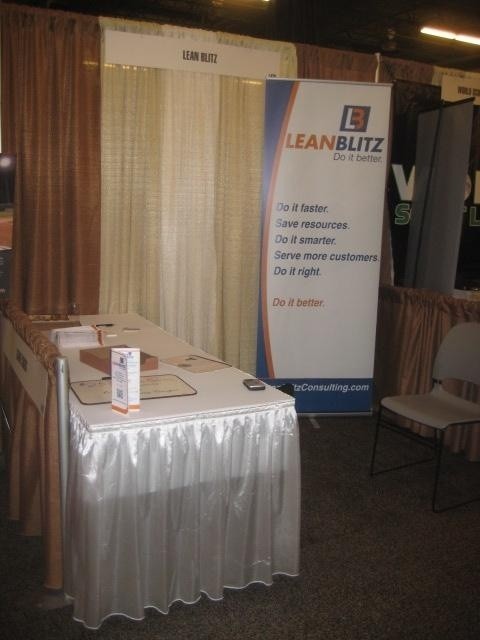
[371,321,480,513]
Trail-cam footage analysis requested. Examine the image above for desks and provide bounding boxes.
[29,312,301,629]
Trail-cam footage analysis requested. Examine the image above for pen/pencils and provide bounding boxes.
[96,324,114,327]
